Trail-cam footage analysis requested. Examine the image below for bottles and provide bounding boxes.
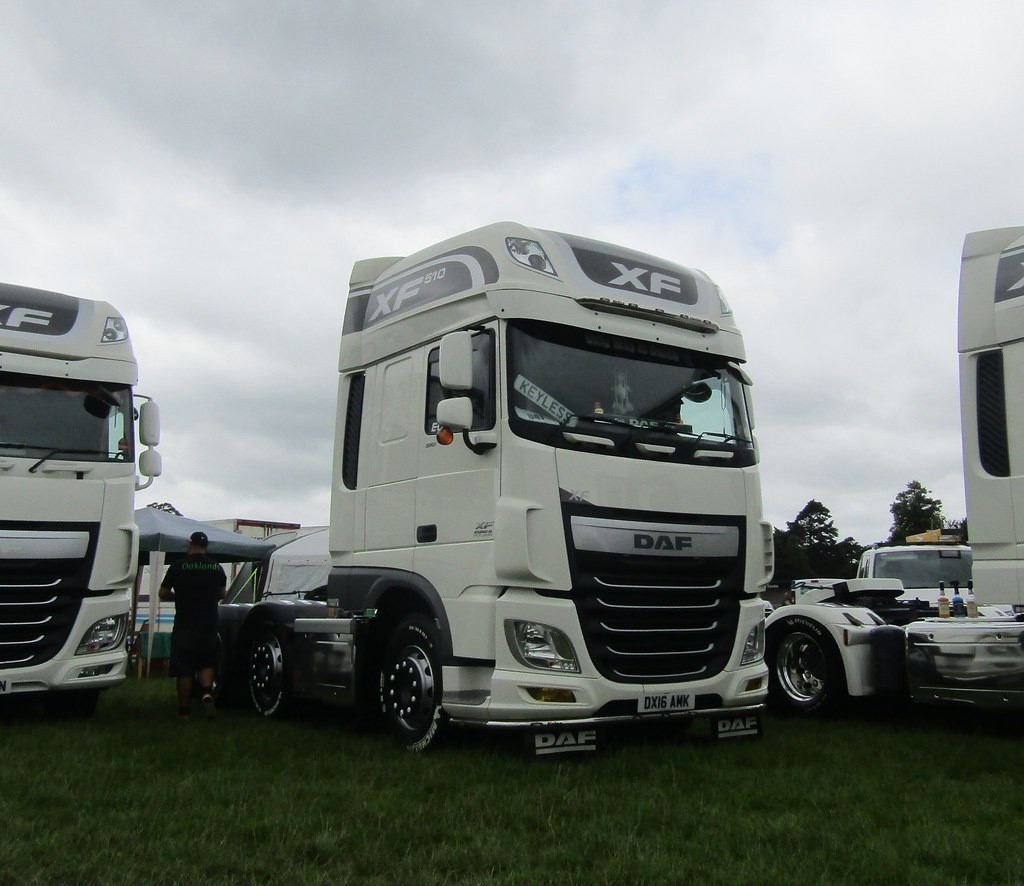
[938,581,950,618]
[592,403,604,414]
[966,581,978,617]
[950,581,965,617]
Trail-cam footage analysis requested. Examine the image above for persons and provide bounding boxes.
[158,532,228,725]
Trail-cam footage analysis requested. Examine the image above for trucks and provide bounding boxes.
[853,527,974,610]
[765,223,1023,719]
[1,277,163,717]
[212,221,785,753]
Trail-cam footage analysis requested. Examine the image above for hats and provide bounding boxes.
[188,532,208,546]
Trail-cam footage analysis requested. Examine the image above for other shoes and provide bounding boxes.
[202,693,216,720]
[180,705,192,721]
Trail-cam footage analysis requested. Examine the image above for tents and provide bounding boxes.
[129,507,276,678]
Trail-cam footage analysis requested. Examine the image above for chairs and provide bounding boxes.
[127,615,173,679]
